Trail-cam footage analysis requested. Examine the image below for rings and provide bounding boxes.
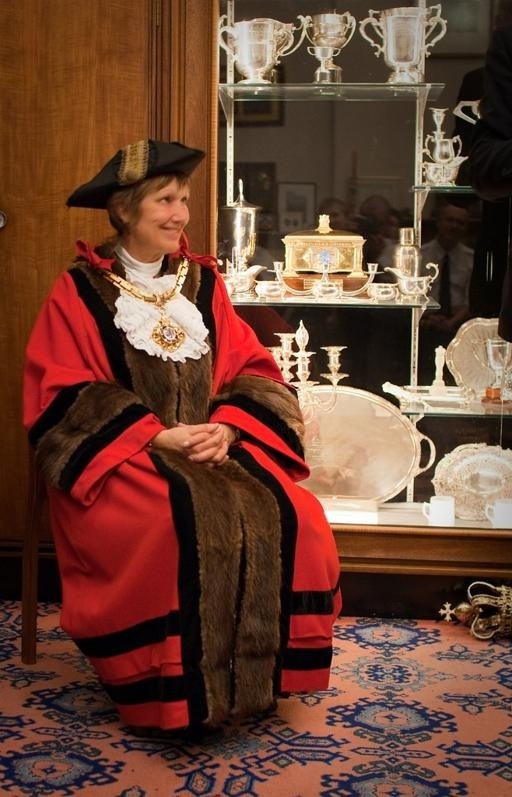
[223,437,232,446]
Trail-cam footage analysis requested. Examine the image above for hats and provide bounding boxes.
[66,139,204,209]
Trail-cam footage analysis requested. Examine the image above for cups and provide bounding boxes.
[484,500,510,528]
[423,495,455,526]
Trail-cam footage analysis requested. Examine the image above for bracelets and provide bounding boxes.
[231,424,241,440]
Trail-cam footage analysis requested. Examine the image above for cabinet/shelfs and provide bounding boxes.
[217,0,512,584]
[0,0,218,560]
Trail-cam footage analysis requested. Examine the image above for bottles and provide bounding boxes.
[397,227,422,294]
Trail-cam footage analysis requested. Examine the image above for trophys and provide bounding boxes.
[421,109,468,186]
[216,4,447,84]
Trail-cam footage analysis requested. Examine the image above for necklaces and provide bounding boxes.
[105,258,189,353]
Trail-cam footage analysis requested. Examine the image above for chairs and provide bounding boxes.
[16,418,61,665]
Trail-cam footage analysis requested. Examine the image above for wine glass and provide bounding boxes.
[471,338,512,405]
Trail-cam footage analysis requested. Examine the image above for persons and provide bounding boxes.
[258,194,472,377]
[22,137,342,738]
[442,2,510,341]
